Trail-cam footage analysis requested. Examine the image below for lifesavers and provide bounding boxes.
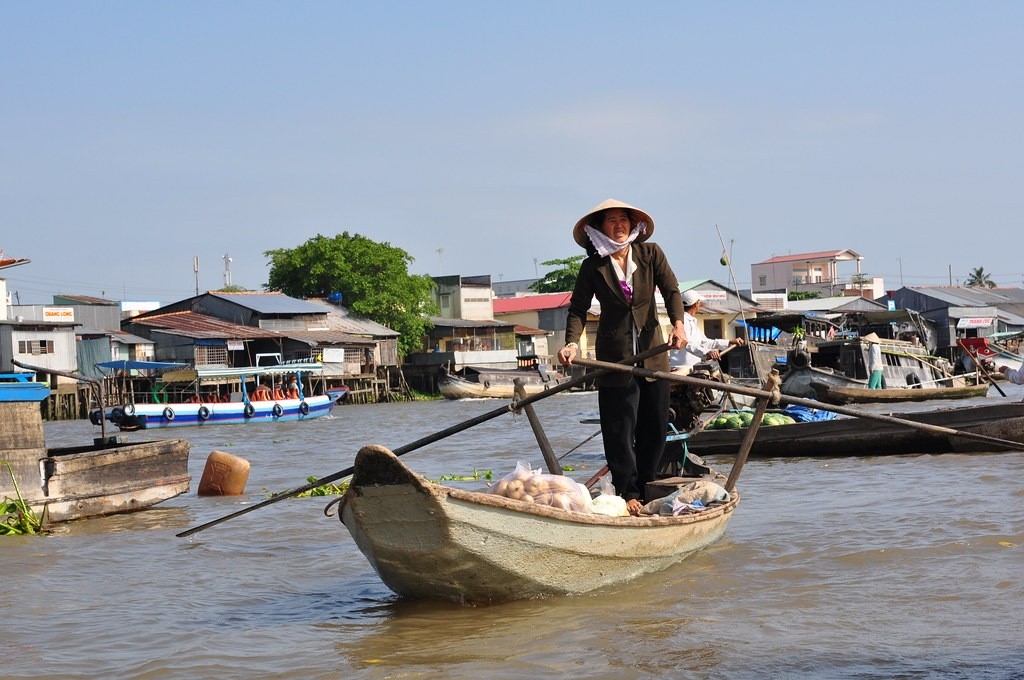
[273,404,284,417]
[123,403,136,417]
[300,401,311,416]
[164,407,175,421]
[93,411,103,425]
[153,384,168,403]
[111,407,123,421]
[244,404,255,417]
[198,407,210,419]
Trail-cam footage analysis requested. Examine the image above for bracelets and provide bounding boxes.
[566,342,578,349]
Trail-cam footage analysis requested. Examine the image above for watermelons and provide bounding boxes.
[705,413,796,430]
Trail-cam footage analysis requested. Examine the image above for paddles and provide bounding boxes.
[176,341,674,541]
[562,351,1023,451]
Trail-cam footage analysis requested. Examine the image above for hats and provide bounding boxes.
[863,333,881,344]
[573,199,655,250]
[682,290,705,306]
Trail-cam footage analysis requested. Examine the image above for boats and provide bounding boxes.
[0,359,193,523]
[685,340,793,407]
[956,330,1024,376]
[779,307,989,403]
[667,399,1024,449]
[91,359,347,428]
[337,446,740,599]
[437,355,572,398]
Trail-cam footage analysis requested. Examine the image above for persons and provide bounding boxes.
[865,332,883,389]
[275,377,286,394]
[256,379,271,391]
[288,375,299,393]
[557,197,688,511]
[670,290,745,373]
[999,361,1024,385]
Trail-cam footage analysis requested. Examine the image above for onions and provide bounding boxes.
[493,471,587,513]
